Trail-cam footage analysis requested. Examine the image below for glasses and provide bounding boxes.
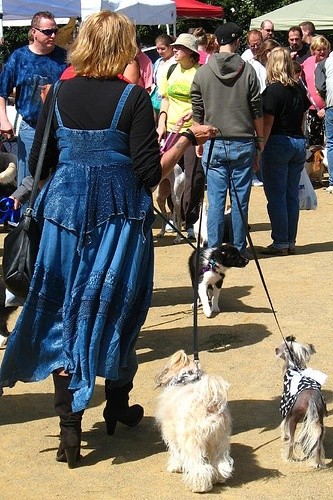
[35,27,59,36]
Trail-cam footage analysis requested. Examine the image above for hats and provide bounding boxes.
[217,22,243,45]
[169,33,202,56]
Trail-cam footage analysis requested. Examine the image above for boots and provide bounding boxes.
[53,369,85,469]
[103,378,144,436]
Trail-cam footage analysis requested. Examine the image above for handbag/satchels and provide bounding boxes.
[298,164,317,210]
[2,207,40,299]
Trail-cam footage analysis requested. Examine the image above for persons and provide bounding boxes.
[0,10,333,468]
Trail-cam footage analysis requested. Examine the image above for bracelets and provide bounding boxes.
[256,137,264,142]
[256,150,261,156]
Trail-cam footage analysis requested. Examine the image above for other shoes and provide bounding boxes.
[165,219,177,231]
[0,331,13,349]
[259,244,296,256]
[186,228,196,243]
[251,179,264,186]
[326,185,333,193]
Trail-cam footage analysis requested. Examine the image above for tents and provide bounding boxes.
[0,0,333,42]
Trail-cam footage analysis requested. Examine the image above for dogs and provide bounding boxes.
[276,340,328,470]
[155,350,234,495]
[156,154,251,244]
[307,144,328,186]
[189,242,248,317]
